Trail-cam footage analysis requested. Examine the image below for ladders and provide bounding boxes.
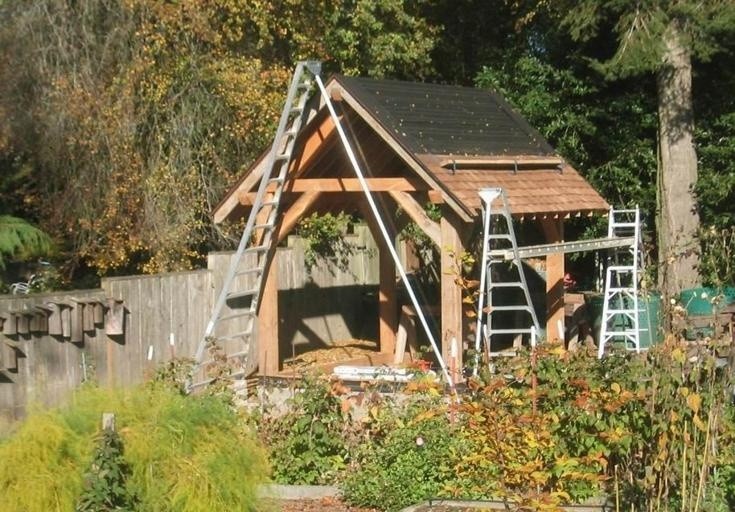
[184,60,456,401]
[597,204,654,357]
[473,187,544,373]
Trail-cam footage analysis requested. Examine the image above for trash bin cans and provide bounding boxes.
[680,288,735,342]
[591,295,663,349]
